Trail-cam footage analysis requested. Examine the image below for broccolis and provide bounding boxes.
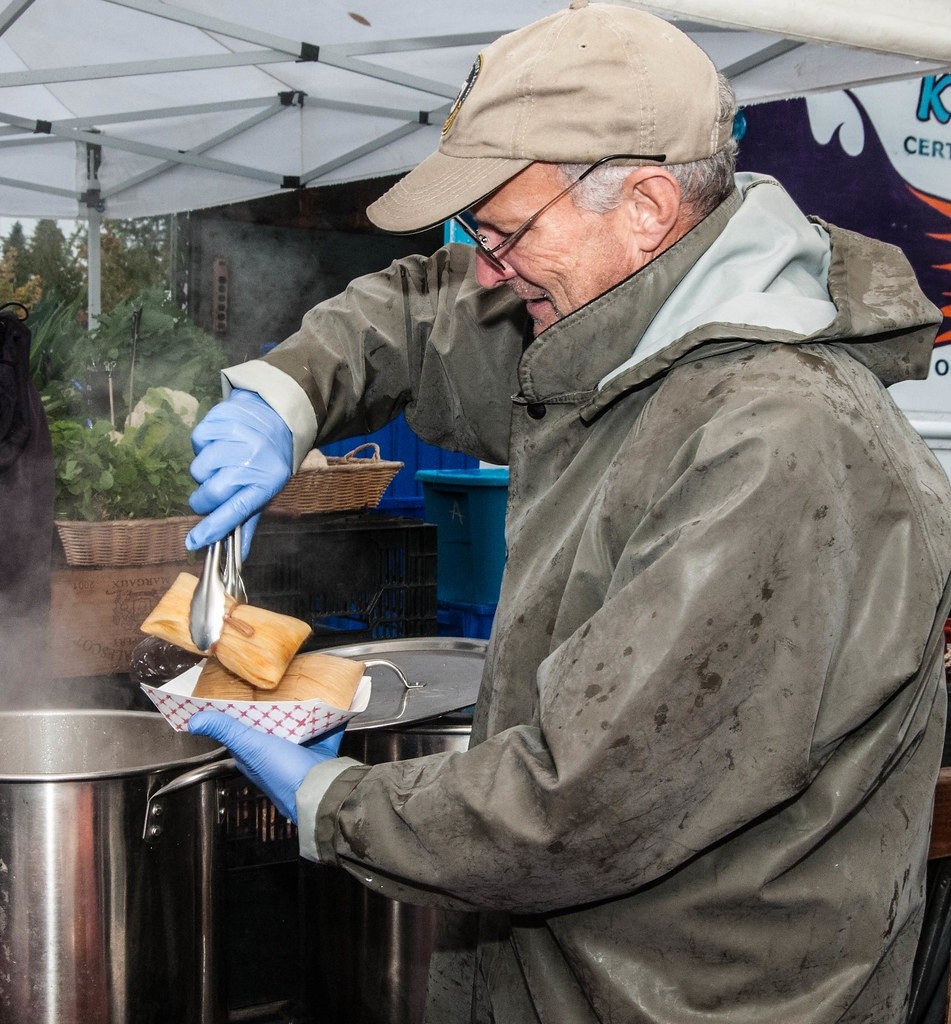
[105,388,332,472]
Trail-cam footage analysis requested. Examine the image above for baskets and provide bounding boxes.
[53,514,212,569]
[264,441,406,517]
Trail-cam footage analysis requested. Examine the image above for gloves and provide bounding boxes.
[187,703,347,829]
[183,387,292,564]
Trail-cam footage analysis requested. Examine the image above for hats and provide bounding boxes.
[364,0,735,237]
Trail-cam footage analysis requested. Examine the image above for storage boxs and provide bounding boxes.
[242,511,439,654]
[41,553,226,681]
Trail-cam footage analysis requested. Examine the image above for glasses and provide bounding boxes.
[455,153,666,271]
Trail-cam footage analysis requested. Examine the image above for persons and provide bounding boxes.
[187,2,951,1024]
